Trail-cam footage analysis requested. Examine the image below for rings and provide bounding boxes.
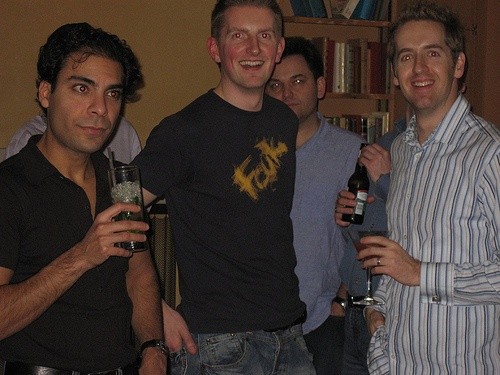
[377,257,381,267]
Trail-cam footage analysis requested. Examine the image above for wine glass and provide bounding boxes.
[347,229,392,305]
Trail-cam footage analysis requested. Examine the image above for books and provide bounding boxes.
[312,35,387,95]
[289,0,389,21]
[322,112,390,145]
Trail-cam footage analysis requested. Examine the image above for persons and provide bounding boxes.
[130,0,318,375]
[333,102,415,375]
[264,35,369,375]
[5,22,146,169]
[0,21,170,375]
[355,4,500,375]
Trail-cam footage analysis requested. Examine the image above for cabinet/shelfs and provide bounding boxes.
[282,1,396,142]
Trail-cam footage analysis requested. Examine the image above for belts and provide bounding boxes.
[4,361,136,375]
[348,295,366,308]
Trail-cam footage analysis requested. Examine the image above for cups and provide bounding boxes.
[108,166,146,252]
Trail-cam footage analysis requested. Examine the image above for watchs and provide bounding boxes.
[140,339,169,355]
[332,296,348,309]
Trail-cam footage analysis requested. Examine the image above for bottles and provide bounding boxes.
[342,143,370,225]
